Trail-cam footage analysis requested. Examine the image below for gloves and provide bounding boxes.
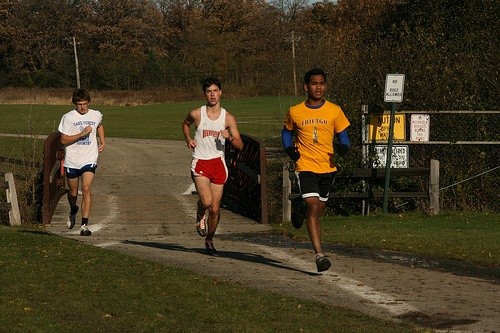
[334,142,348,156]
[287,146,300,162]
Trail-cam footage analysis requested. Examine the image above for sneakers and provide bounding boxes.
[67,206,79,229]
[291,198,304,229]
[196,209,207,236]
[80,225,91,236]
[316,255,331,272]
[205,238,215,252]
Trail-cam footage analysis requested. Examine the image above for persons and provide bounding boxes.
[281,68,354,272]
[58,88,106,235]
[182,76,243,254]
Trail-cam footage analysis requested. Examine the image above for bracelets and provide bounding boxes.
[230,136,234,143]
